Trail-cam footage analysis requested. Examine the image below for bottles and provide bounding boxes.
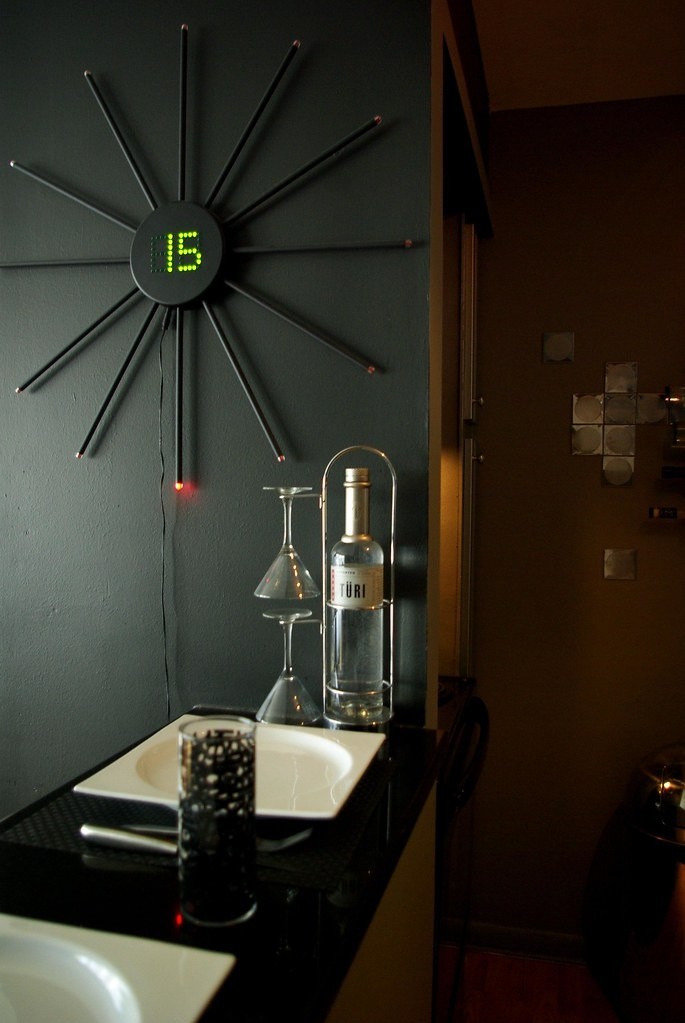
[331,469,384,720]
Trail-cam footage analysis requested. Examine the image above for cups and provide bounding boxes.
[179,715,255,927]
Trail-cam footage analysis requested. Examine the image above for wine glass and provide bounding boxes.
[253,486,320,600]
[256,608,321,726]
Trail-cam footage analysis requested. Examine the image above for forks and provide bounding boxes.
[119,822,313,851]
